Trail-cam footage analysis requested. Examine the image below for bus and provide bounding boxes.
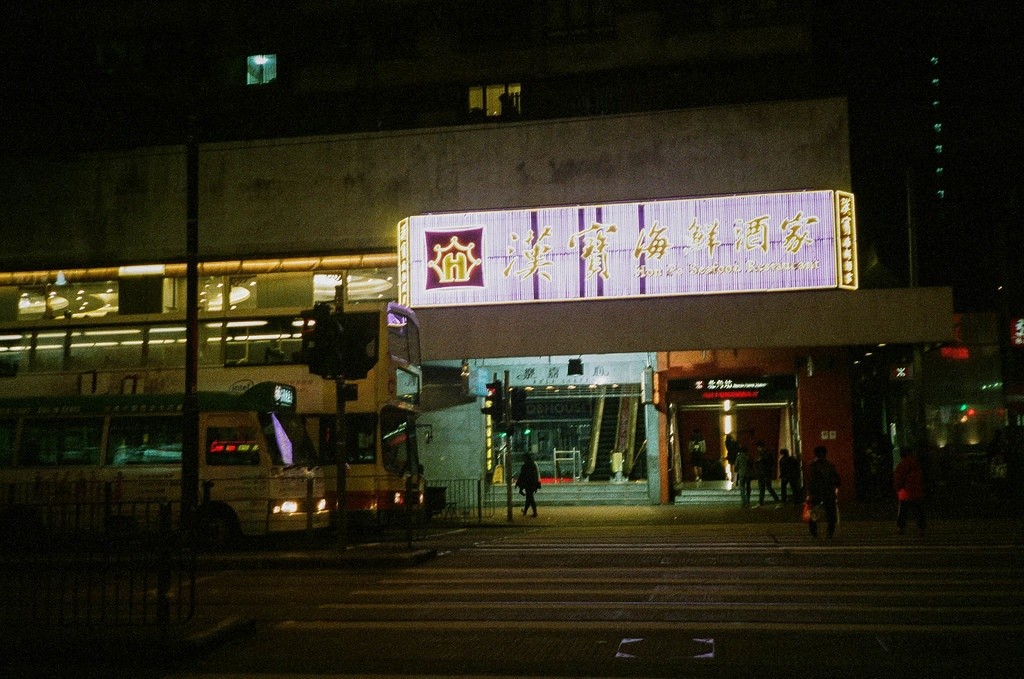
[417,364,489,516]
[0,302,433,534]
[0,382,332,546]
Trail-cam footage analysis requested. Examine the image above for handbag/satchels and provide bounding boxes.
[802,501,810,521]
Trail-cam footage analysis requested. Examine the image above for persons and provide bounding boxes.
[515,454,540,518]
[778,449,802,501]
[269,339,285,357]
[804,447,840,539]
[687,429,705,483]
[725,434,781,504]
[893,448,928,537]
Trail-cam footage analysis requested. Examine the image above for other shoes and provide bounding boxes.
[530,514,538,518]
[775,503,782,510]
[521,508,526,515]
[751,504,764,509]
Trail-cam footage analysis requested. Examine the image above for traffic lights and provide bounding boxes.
[300,309,332,373]
[482,380,503,422]
